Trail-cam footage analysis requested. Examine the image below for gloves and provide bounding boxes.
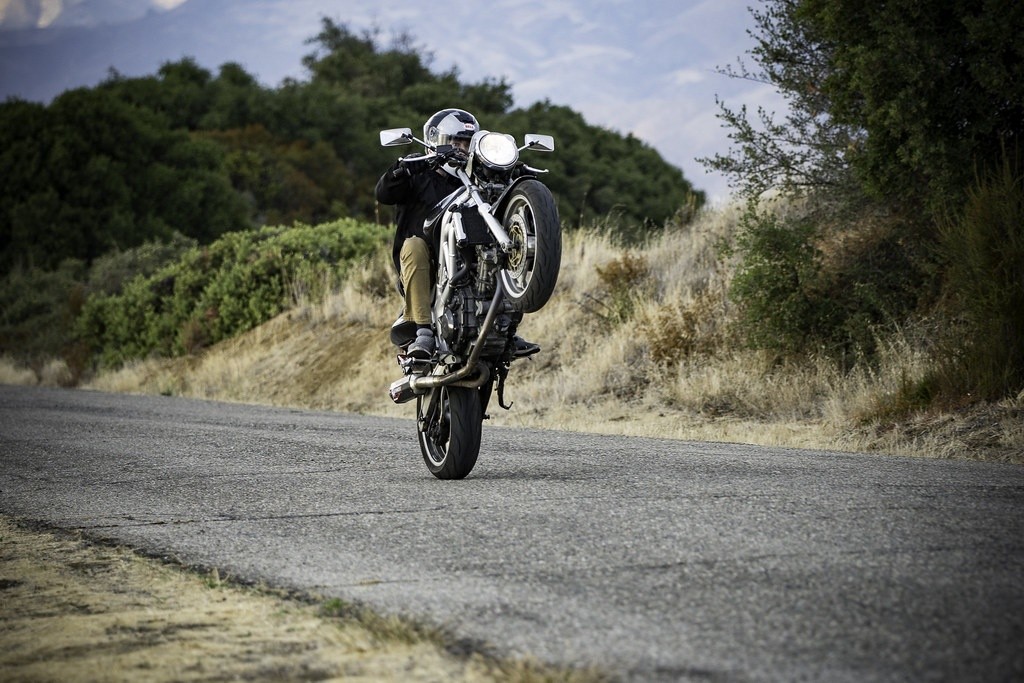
[515,162,539,177]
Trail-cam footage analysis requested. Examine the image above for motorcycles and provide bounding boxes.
[377,122,564,483]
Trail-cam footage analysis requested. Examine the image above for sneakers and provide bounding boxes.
[511,336,541,357]
[407,328,434,358]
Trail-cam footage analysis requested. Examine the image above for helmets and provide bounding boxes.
[422,108,479,179]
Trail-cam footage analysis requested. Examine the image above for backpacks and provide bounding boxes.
[399,154,427,176]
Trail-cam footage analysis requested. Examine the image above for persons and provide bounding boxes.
[373,107,539,372]
